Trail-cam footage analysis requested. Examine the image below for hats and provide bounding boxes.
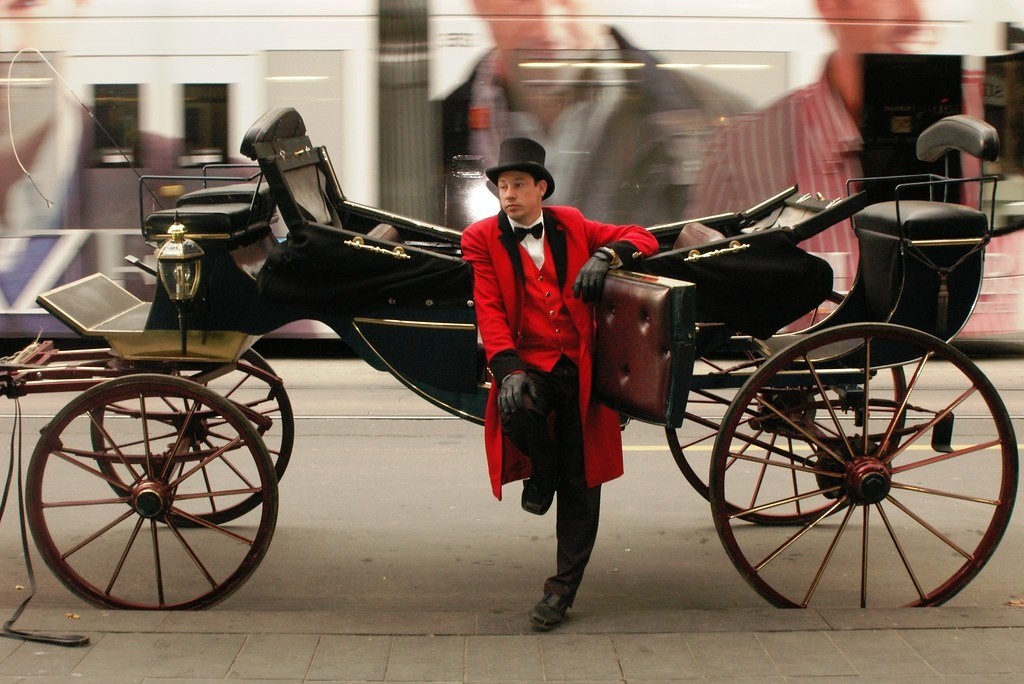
[485,138,555,201]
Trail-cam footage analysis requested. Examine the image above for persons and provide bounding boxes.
[461,137,658,628]
[0,1,315,337]
[430,0,750,230]
[692,1,1024,339]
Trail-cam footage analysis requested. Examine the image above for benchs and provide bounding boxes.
[138,105,308,311]
[674,222,724,251]
[815,114,1000,348]
[369,224,400,245]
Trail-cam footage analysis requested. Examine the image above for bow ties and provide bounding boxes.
[514,221,543,243]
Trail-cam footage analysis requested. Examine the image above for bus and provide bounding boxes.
[0,0,1024,356]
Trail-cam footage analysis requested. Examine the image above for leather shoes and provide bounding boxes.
[529,593,567,629]
[521,470,555,516]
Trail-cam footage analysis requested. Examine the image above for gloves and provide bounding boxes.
[571,247,613,304]
[498,372,538,415]
